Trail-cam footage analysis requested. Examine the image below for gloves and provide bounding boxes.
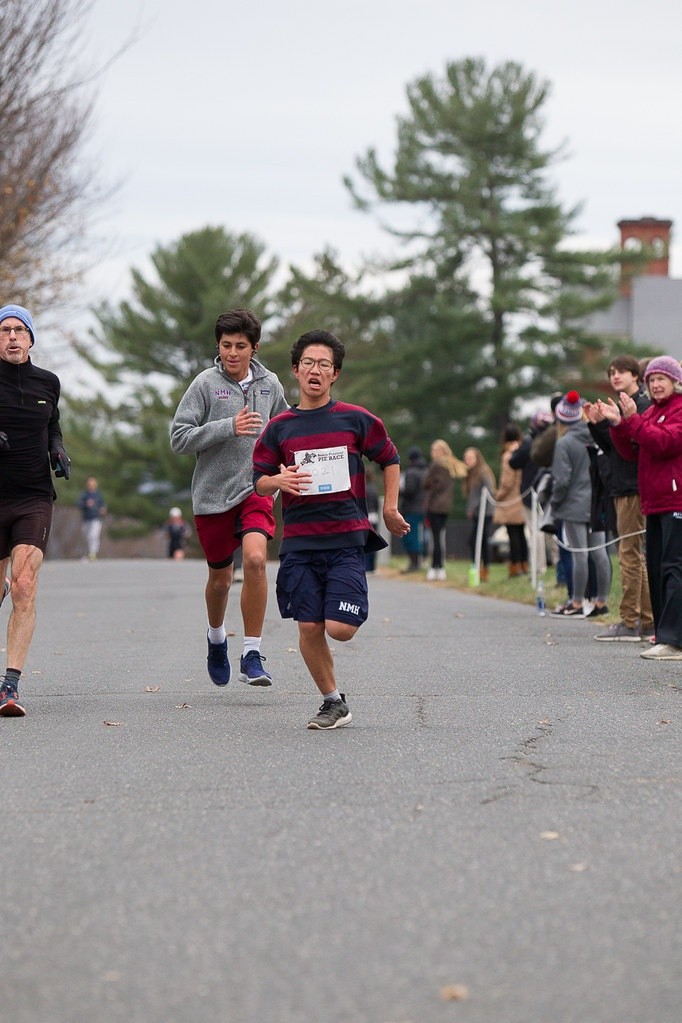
[50,446,71,480]
[0,432,11,448]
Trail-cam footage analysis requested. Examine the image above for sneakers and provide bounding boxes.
[594,622,656,642]
[307,693,352,730]
[0,577,12,608]
[238,650,273,687]
[206,628,232,686]
[0,674,27,717]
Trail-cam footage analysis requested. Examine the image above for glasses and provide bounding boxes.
[0,325,29,335]
[299,357,334,371]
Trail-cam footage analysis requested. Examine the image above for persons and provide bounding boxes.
[167,307,297,688]
[166,507,193,562]
[508,382,616,618]
[0,302,72,717]
[461,446,496,582]
[419,439,470,580]
[250,330,412,731]
[580,354,659,643]
[493,427,531,580]
[596,355,682,662]
[79,477,107,562]
[392,446,431,574]
[360,463,381,571]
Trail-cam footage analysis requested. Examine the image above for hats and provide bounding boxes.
[531,412,553,433]
[644,356,682,384]
[555,392,583,422]
[407,448,427,467]
[0,304,35,349]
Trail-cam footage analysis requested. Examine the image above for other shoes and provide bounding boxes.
[586,603,610,620]
[640,642,682,660]
[549,603,585,618]
[508,563,522,577]
[480,567,489,580]
[521,561,530,575]
[400,557,418,575]
[438,569,445,580]
[427,568,436,580]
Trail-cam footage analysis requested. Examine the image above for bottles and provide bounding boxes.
[536,580,546,616]
[467,564,476,588]
